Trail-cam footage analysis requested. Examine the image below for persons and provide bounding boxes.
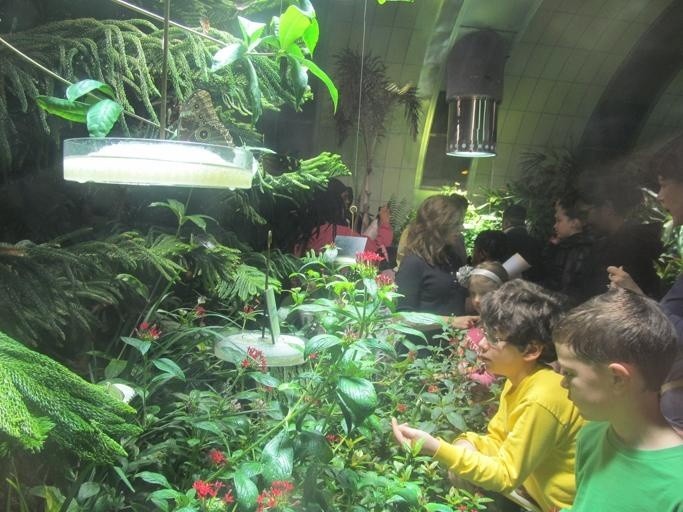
[291,177,393,263]
[390,278,585,512]
[549,288,683,511]
[397,132,683,421]
